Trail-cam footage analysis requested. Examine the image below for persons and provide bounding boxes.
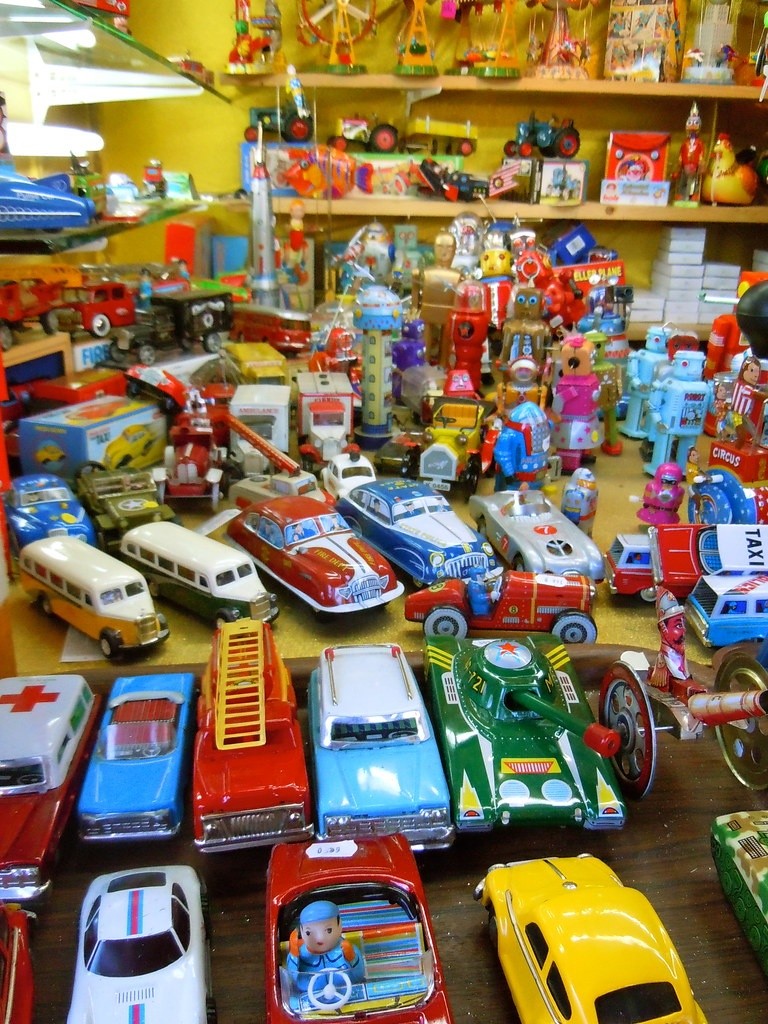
[407,231,709,481]
[646,585,693,688]
[287,900,366,993]
[686,447,706,509]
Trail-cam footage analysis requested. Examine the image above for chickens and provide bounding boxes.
[701,133,760,206]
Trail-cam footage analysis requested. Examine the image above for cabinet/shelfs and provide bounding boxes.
[224,71,767,346]
[0,1,239,253]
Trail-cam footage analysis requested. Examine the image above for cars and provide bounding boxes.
[64,863,221,1024]
[262,832,455,1024]
[710,808,768,975]
[0,96,768,908]
[470,853,707,1024]
[0,904,36,1023]
